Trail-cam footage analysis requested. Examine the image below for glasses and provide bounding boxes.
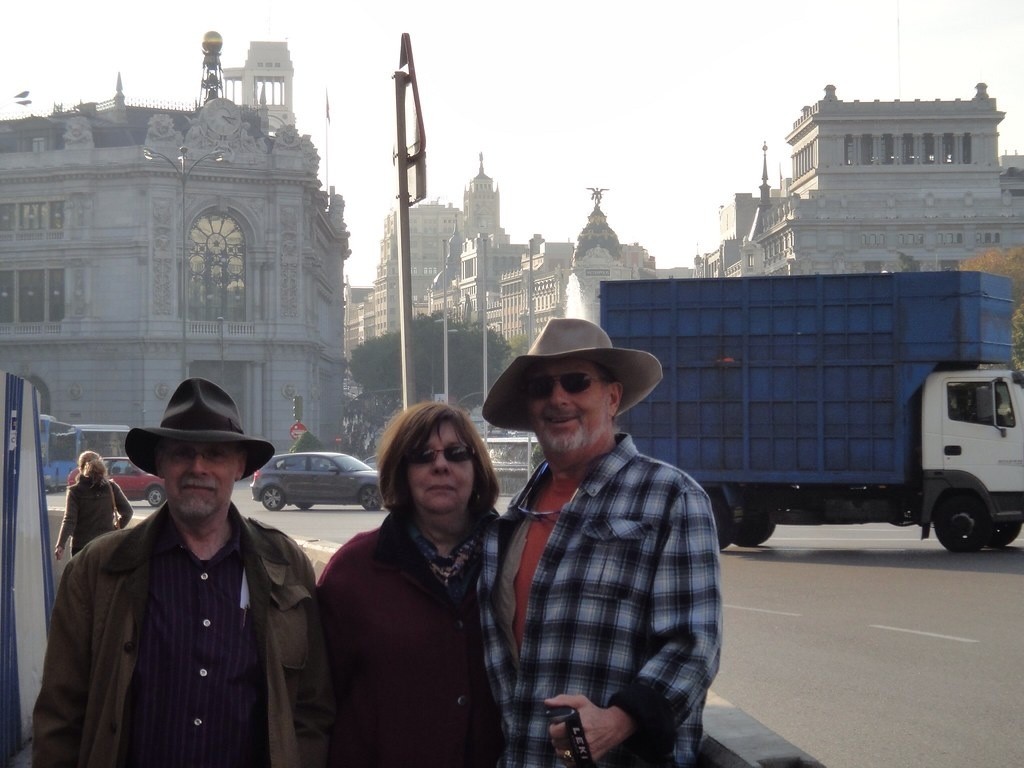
[522,372,610,399]
[166,444,230,464]
[408,444,473,464]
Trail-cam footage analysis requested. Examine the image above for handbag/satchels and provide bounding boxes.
[107,481,121,530]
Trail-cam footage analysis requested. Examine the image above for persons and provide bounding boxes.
[308,402,507,768]
[28,377,338,768]
[54,450,134,562]
[474,317,721,768]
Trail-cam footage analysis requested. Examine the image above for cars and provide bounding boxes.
[250,452,384,512]
[68,457,167,507]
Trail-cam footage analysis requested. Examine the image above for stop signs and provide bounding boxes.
[334,437,343,445]
[290,423,307,440]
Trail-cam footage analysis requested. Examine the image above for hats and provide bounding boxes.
[482,319,663,432]
[125,377,275,481]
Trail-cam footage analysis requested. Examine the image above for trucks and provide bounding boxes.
[601,271,1024,554]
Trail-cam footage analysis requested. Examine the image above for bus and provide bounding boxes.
[37,414,81,493]
